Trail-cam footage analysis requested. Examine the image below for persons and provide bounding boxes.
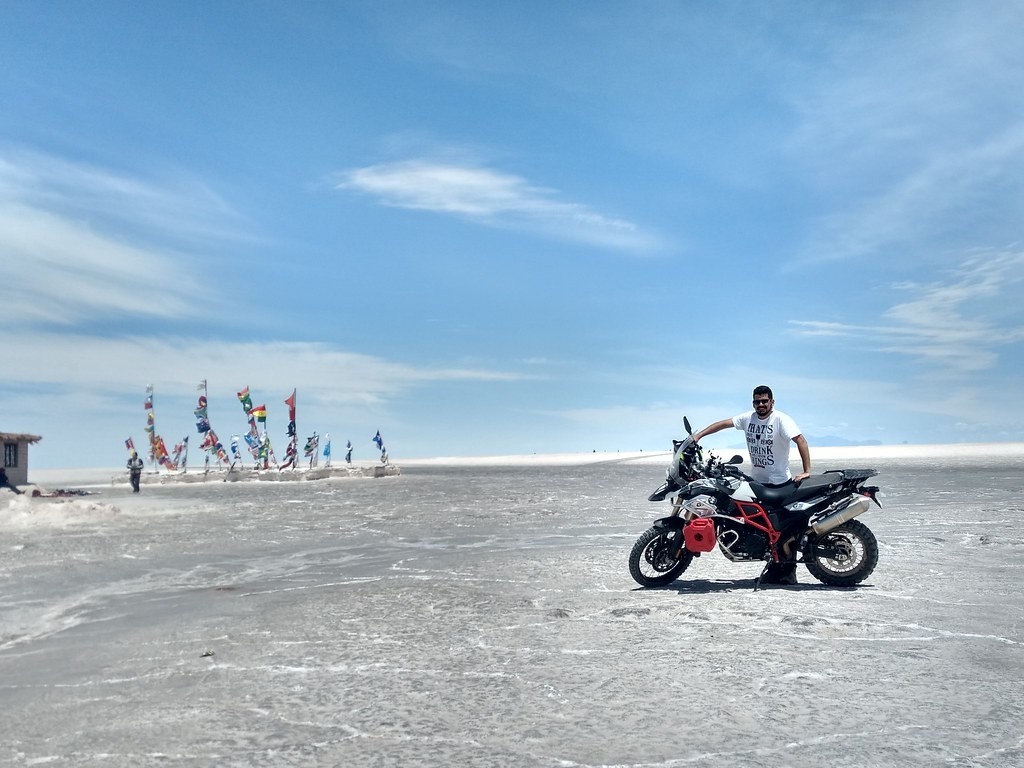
[692,385,811,586]
[127,452,143,493]
[0,468,25,494]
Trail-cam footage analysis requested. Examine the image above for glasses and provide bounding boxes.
[752,398,769,404]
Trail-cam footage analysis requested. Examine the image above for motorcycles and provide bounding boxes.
[626,415,885,590]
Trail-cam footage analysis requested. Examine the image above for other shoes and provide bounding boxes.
[754,563,779,585]
[772,563,797,585]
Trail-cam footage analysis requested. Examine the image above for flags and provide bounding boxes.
[125,379,388,470]
